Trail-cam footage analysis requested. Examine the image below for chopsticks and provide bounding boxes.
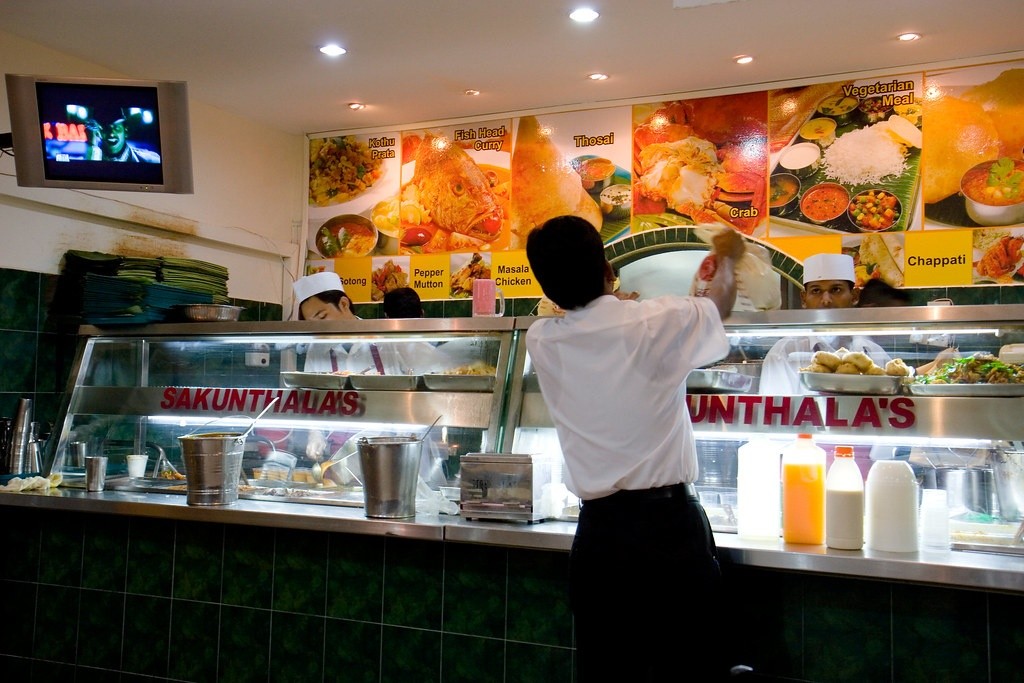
[461,502,526,509]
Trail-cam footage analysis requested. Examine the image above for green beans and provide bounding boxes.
[358,165,364,179]
[853,198,899,226]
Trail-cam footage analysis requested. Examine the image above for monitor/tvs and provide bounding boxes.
[3,72,195,194]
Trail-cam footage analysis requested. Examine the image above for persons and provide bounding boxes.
[523,215,753,683]
[83,107,160,163]
[382,286,425,318]
[860,276,900,306]
[760,254,899,481]
[293,270,464,494]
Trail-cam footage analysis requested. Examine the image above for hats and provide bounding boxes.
[802,253,856,286]
[293,272,345,306]
[93,104,126,124]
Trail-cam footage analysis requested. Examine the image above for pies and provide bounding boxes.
[807,351,909,376]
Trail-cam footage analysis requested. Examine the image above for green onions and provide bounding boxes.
[601,190,632,204]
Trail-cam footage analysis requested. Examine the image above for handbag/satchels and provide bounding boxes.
[690,222,782,312]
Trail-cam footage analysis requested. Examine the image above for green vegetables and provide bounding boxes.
[913,355,1014,384]
[333,137,346,149]
[771,182,787,201]
[986,157,1022,189]
[321,227,350,255]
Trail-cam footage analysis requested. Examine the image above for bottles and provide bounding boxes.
[781,433,826,545]
[824,445,864,550]
[864,460,919,553]
[736,437,780,540]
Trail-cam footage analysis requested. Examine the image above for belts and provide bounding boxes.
[582,483,698,512]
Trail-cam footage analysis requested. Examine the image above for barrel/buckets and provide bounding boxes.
[922,465,1001,517]
[355,433,425,518]
[177,415,254,506]
[987,446,1024,521]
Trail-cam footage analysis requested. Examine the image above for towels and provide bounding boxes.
[0,472,64,494]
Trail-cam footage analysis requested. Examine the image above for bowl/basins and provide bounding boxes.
[798,117,837,150]
[799,182,851,226]
[858,96,893,124]
[816,93,859,128]
[575,157,616,194]
[847,189,904,233]
[169,304,248,322]
[313,212,379,259]
[770,173,802,217]
[369,196,398,256]
[308,132,400,221]
[779,142,822,179]
[440,486,461,500]
[402,148,510,186]
[958,158,1024,226]
[599,183,632,220]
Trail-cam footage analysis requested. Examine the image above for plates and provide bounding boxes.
[246,478,337,489]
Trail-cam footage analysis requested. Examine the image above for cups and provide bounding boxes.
[920,488,952,554]
[472,279,505,318]
[9,398,43,474]
[70,441,87,467]
[125,454,149,477]
[85,456,109,492]
[697,491,738,508]
[252,466,317,482]
